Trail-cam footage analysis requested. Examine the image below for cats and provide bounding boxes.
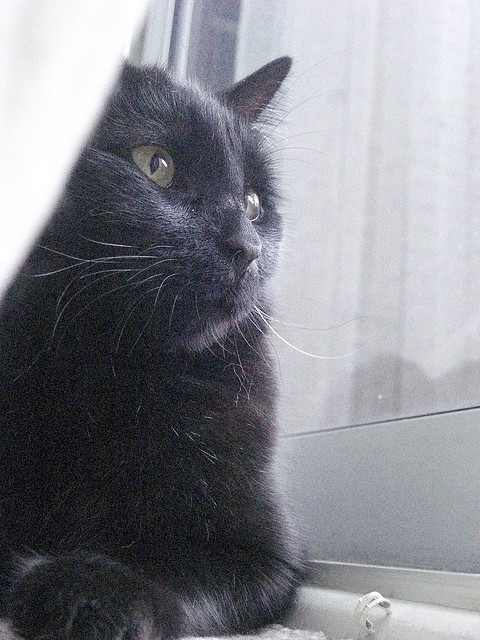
[0,56,302,639]
